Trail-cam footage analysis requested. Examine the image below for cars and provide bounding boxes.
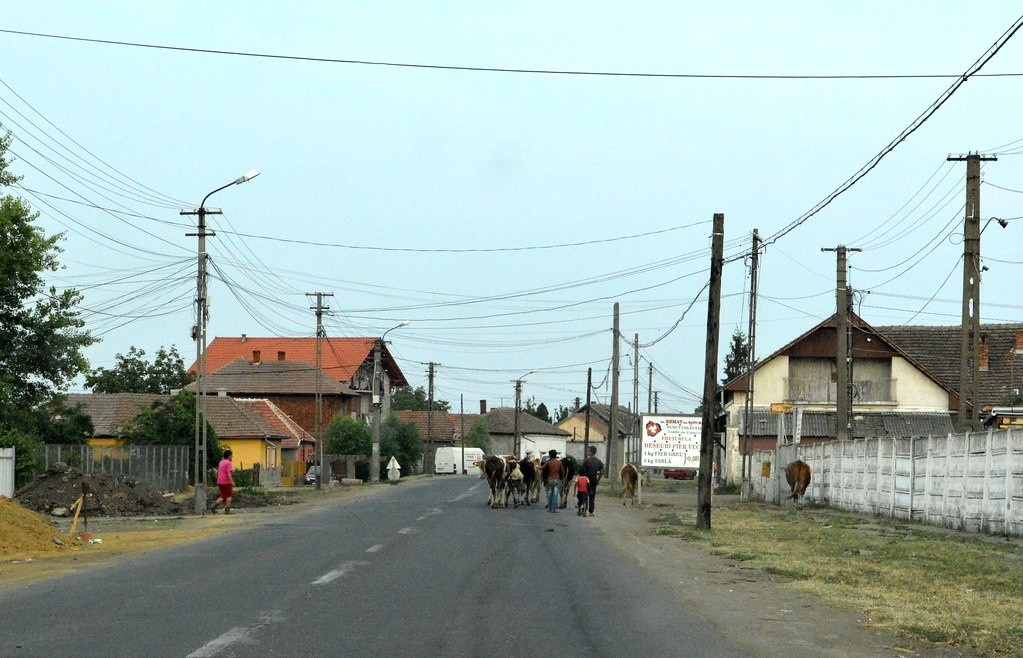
[304,465,321,484]
[663,468,695,481]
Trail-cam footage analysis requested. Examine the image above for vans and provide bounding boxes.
[434,447,486,476]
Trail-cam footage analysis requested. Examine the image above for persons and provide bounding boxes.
[583,446,604,517]
[537,449,565,514]
[573,468,592,517]
[211,450,236,514]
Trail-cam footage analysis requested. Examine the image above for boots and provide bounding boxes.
[224,506,235,514]
[210,502,219,515]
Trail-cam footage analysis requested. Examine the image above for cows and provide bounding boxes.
[620,463,648,506]
[556,457,574,509]
[785,460,811,509]
[473,455,549,509]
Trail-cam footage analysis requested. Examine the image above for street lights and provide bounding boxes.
[189,171,264,513]
[512,370,538,461]
[372,320,412,484]
